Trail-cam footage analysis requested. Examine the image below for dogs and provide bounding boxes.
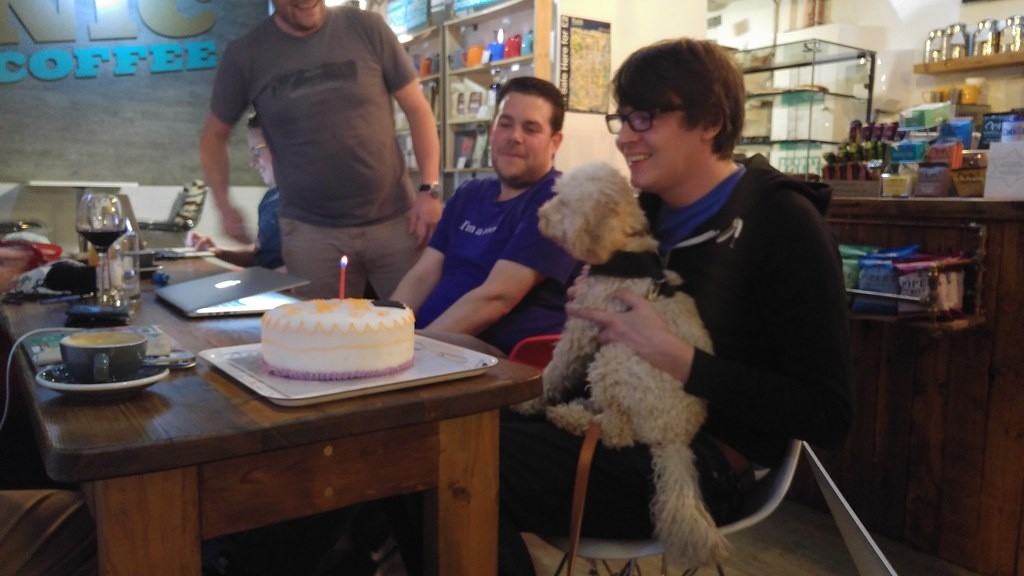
[536,161,730,568]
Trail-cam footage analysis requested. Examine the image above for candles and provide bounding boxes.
[338,254,348,300]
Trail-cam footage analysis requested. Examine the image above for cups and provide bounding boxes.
[107,232,140,302]
[59,332,147,382]
[449,30,533,68]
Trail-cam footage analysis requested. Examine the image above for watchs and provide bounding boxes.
[419,182,441,199]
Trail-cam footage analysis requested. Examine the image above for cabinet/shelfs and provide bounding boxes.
[733,38,876,183]
[387,0,554,205]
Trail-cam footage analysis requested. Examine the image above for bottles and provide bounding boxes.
[923,14,1024,62]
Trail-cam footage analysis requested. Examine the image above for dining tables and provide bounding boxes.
[0,248,543,576]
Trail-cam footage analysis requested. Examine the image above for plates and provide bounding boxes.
[36,366,169,395]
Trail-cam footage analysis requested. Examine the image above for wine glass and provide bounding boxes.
[75,196,127,305]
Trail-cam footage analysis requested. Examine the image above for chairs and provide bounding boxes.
[535,439,806,576]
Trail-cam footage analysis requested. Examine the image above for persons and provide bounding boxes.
[392,38,846,576]
[192,112,286,271]
[215,77,588,575]
[200,0,444,304]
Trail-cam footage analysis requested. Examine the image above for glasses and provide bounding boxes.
[251,141,268,157]
[605,104,683,134]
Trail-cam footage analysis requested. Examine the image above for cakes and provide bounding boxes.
[260,298,415,381]
